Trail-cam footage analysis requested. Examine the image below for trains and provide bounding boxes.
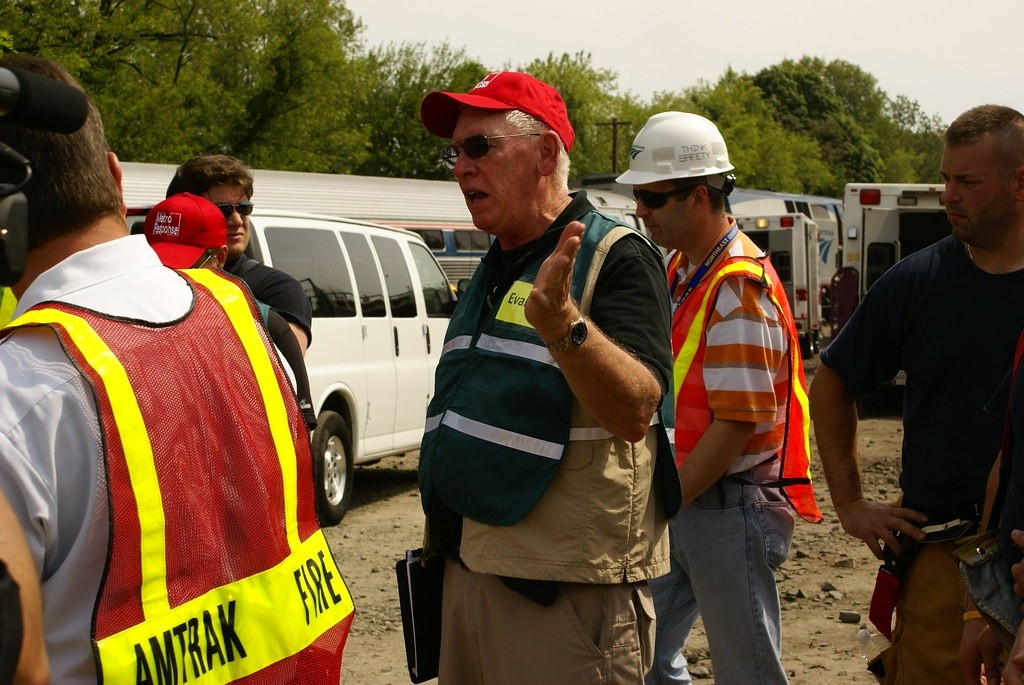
[102,162,842,295]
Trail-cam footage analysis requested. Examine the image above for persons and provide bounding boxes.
[808,104,1024,685]
[414,71,675,685]
[614,111,825,685]
[143,191,317,431]
[0,50,355,685]
[165,155,313,358]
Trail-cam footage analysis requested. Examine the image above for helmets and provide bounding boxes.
[615,111,735,185]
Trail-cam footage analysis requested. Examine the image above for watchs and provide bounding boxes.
[544,312,588,353]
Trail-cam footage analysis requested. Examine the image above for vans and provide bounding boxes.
[127,211,472,526]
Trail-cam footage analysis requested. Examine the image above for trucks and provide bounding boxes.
[737,213,821,360]
[843,183,947,305]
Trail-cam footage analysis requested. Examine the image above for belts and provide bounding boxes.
[757,454,777,466]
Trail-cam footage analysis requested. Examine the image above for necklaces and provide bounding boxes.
[967,244,974,263]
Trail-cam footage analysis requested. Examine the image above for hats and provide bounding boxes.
[419,72,575,154]
[145,192,228,269]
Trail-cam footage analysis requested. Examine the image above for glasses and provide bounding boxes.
[442,133,542,170]
[633,188,695,208]
[216,201,255,218]
[191,251,214,269]
